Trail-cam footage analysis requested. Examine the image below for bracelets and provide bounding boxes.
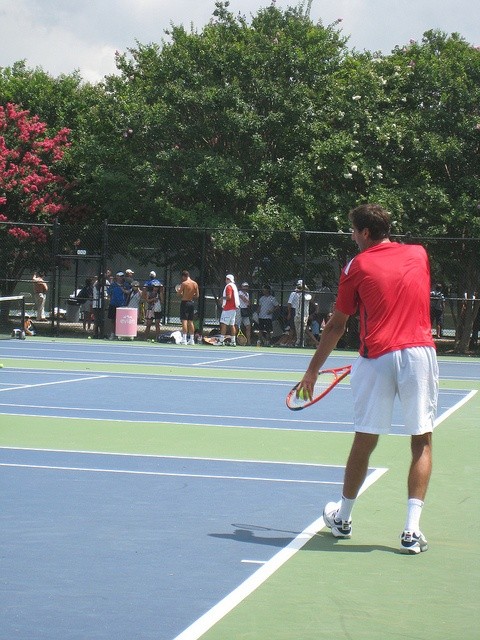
[222,297,226,300]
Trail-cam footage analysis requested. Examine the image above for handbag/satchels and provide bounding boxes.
[146,310,155,319]
[241,308,250,317]
[24,320,37,336]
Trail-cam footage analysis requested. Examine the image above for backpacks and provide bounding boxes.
[78,290,88,303]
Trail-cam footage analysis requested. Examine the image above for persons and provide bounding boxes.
[235,282,251,344]
[142,270,165,336]
[186,311,199,341]
[124,269,135,285]
[287,279,312,347]
[251,305,259,326]
[119,280,148,321]
[32,270,48,321]
[145,282,164,340]
[429,283,446,338]
[77,279,94,333]
[212,273,240,347]
[296,203,440,554]
[106,267,114,283]
[105,272,132,340]
[176,269,199,345]
[92,271,105,339]
[463,291,476,306]
[327,312,334,321]
[305,301,319,348]
[312,280,336,331]
[345,306,360,349]
[257,285,279,343]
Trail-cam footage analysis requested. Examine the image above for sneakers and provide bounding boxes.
[230,342,236,346]
[400,530,428,554]
[187,339,194,345]
[323,501,352,539]
[180,340,187,345]
[41,319,48,321]
[213,339,223,345]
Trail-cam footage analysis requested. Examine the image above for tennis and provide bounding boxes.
[0,363,3,369]
[298,387,310,400]
[88,336,91,339]
[152,339,155,343]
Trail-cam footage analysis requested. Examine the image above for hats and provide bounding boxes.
[150,271,156,278]
[226,274,235,283]
[116,272,124,276]
[242,282,249,286]
[126,269,134,274]
